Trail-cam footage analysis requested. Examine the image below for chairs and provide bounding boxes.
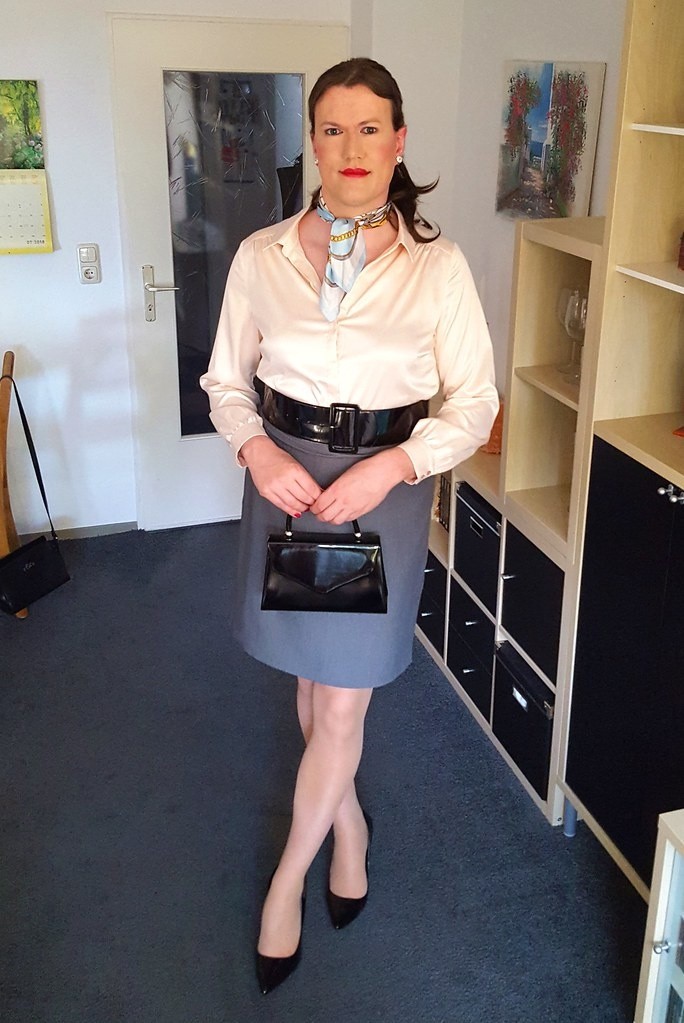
[0,351,29,619]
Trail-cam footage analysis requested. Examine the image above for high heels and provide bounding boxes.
[256,863,308,995]
[327,808,374,931]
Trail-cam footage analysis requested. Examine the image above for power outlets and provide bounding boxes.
[77,242,101,284]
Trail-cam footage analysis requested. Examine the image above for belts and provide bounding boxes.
[252,375,429,454]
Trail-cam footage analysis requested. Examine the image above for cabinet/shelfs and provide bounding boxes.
[411,0,684,897]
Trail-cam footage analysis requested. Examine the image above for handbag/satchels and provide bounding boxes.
[259,514,390,615]
[0,536,71,617]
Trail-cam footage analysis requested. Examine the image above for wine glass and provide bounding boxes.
[554,282,588,385]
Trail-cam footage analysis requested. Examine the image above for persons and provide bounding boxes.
[201,56,499,995]
